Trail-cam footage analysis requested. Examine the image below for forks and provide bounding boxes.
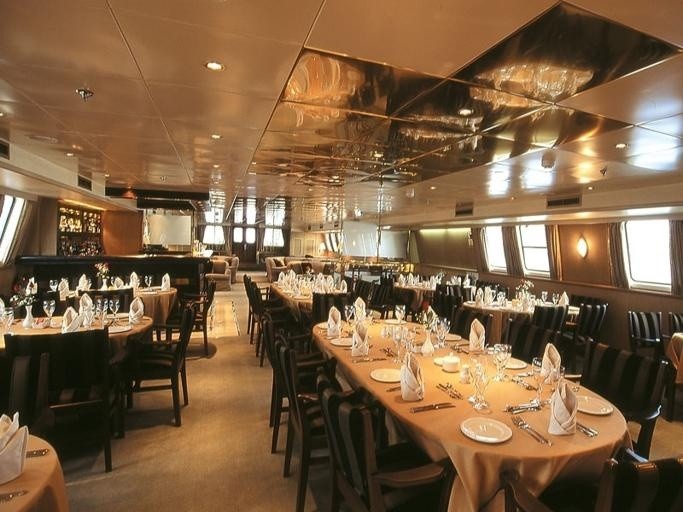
[510,416,552,448]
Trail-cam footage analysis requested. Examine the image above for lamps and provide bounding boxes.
[575,231,588,260]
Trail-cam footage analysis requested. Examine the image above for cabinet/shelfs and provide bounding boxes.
[59,203,104,255]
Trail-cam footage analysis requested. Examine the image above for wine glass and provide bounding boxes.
[475,285,559,310]
[466,358,564,411]
[40,298,120,329]
[281,273,333,298]
[345,303,450,365]
[49,274,153,295]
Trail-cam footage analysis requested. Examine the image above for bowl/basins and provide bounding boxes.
[202,250,213,257]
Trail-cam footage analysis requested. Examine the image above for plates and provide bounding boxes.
[459,416,513,446]
[493,357,527,370]
[319,322,341,329]
[383,318,406,325]
[574,395,614,416]
[329,338,354,346]
[432,357,443,366]
[438,333,462,341]
[368,366,401,383]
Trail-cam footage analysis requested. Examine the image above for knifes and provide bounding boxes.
[435,383,463,400]
[409,402,455,416]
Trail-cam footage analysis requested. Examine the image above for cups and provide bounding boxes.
[442,356,460,373]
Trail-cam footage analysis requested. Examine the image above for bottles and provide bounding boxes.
[63,239,98,256]
[461,365,471,385]
